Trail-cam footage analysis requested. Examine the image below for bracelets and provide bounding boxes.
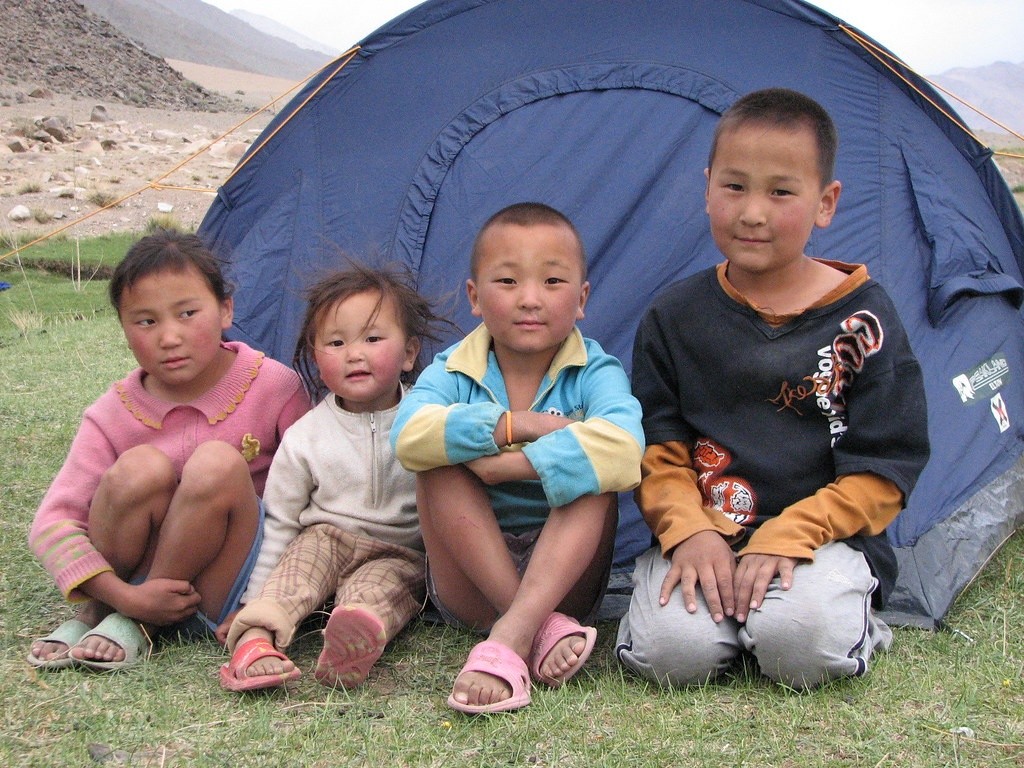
[505,410,513,447]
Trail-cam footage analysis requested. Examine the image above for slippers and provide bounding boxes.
[524,610,598,689]
[68,611,151,669]
[220,638,301,691]
[448,642,531,712]
[314,604,388,691]
[27,620,90,668]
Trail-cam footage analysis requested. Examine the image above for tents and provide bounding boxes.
[191,0,1022,627]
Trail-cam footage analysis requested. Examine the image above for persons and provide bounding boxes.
[610,84,933,690]
[211,231,461,692]
[22,231,285,670]
[384,200,647,712]
